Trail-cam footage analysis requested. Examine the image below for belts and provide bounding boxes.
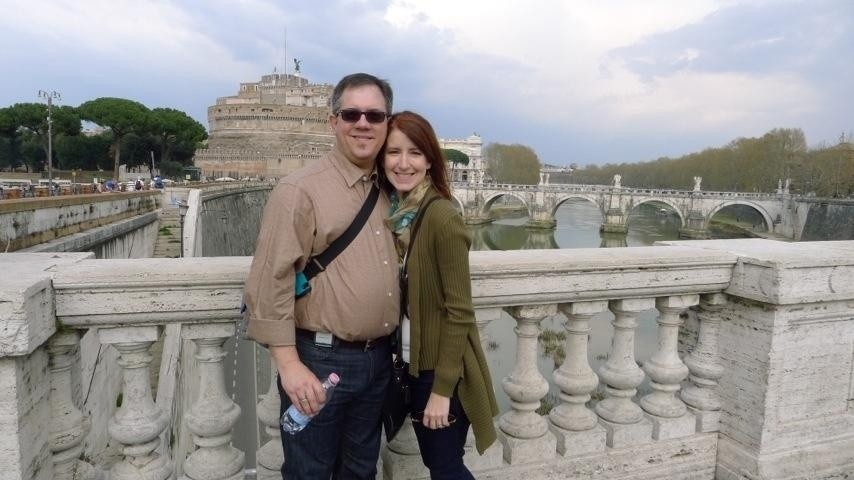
[294,325,399,354]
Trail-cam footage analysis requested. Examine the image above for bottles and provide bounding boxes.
[280,369,340,436]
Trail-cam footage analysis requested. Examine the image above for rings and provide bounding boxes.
[437,422,442,427]
[299,396,306,402]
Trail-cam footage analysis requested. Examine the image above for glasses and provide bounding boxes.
[408,409,457,428]
[333,108,388,124]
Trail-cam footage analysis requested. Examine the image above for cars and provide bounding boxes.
[215,177,234,182]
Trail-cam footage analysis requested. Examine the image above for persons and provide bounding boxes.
[239,70,406,479]
[373,110,502,480]
[52,175,166,197]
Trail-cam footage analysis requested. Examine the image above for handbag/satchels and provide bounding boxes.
[381,359,414,446]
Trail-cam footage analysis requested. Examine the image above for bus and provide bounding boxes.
[0,178,73,192]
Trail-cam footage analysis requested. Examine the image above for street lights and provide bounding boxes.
[37,89,61,197]
